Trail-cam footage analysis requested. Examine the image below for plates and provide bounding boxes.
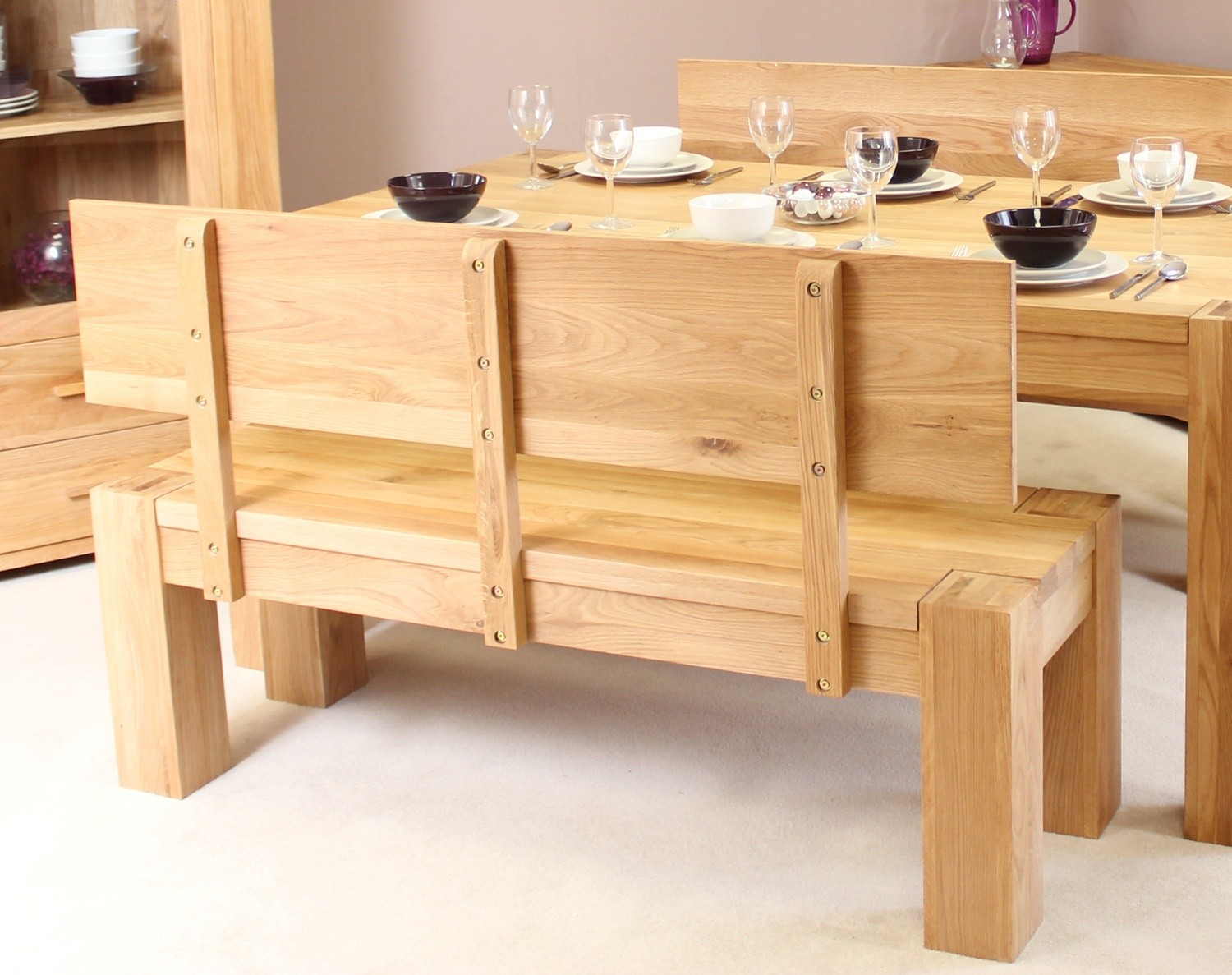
[362,151,1232,290]
[0,85,40,119]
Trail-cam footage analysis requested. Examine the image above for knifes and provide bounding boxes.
[1109,264,1155,298]
[1052,194,1083,208]
[545,170,578,180]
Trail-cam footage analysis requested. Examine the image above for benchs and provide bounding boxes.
[69,60,1231,966]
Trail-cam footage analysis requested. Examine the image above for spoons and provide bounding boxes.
[537,161,578,173]
[1042,184,1072,205]
[1134,261,1188,301]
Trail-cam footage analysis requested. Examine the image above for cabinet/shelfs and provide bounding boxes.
[0,0,276,576]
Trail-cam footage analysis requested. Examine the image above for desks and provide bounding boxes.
[278,150,1232,847]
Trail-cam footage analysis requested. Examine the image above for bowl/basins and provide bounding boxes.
[56,28,158,105]
[386,127,1198,268]
[0,25,8,80]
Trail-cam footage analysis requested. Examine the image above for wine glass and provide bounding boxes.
[508,83,555,191]
[583,114,636,231]
[1010,103,1061,208]
[835,125,898,253]
[1129,136,1186,266]
[746,95,795,186]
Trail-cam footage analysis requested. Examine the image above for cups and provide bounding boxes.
[979,0,1076,70]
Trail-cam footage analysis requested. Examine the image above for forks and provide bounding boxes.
[953,180,997,202]
[1209,202,1232,213]
[686,166,744,185]
